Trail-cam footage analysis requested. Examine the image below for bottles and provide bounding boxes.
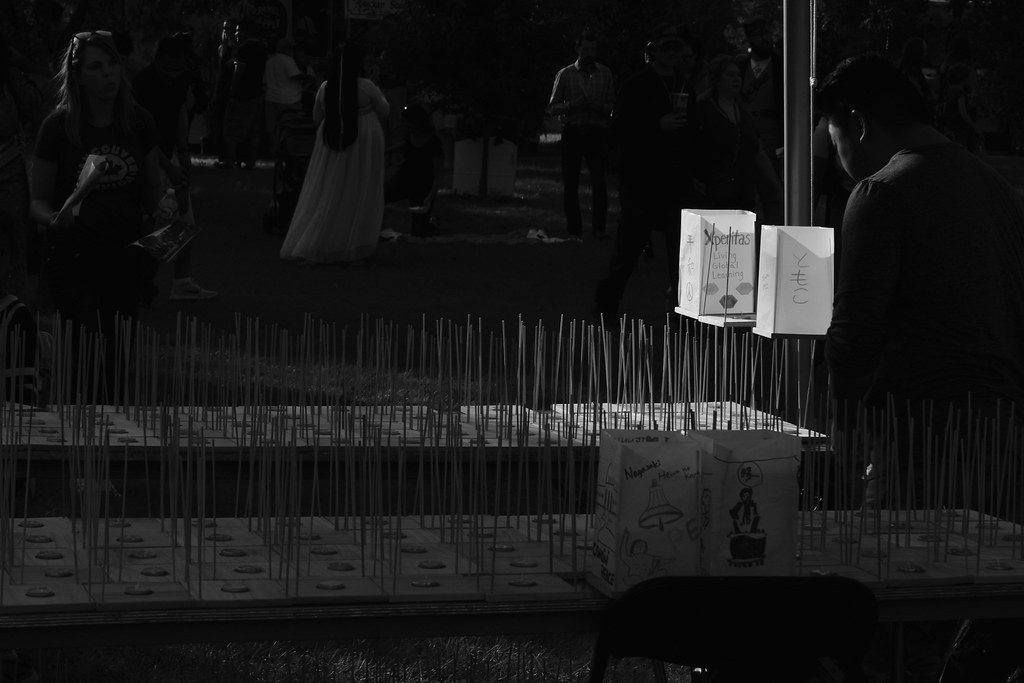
[153,188,178,231]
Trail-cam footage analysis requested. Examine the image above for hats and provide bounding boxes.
[645,22,685,47]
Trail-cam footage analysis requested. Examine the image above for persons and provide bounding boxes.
[1,19,445,408]
[551,29,1024,522]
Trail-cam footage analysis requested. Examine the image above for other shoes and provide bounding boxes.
[214,160,234,169]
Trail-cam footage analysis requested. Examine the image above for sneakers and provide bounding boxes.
[169,276,218,300]
[590,310,619,327]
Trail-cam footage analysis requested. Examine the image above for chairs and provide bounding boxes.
[588,576,878,683]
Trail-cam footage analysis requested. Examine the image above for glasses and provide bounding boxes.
[70,30,113,52]
